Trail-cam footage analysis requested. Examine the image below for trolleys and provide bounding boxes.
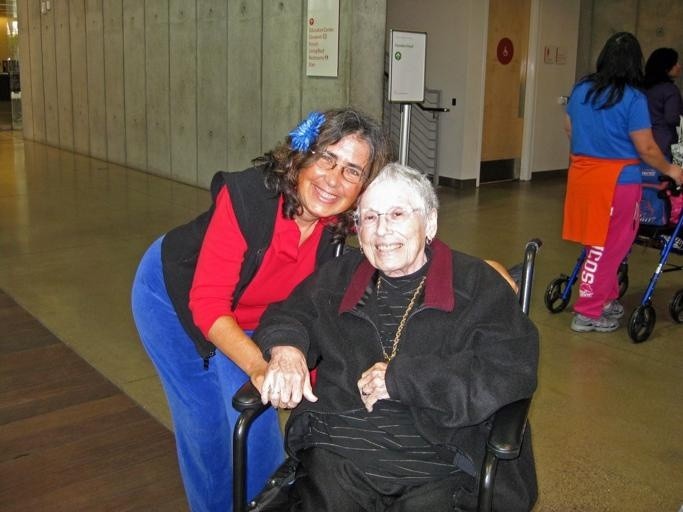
[544,173,683,344]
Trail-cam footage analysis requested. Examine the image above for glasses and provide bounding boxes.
[355,207,424,228]
[308,147,368,185]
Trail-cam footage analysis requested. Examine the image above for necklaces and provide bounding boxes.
[365,274,434,364]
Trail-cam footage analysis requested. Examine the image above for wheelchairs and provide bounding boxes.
[230,235,542,512]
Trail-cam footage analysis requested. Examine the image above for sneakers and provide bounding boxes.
[571,314,620,333]
[602,302,624,318]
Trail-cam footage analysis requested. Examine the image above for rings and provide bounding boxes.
[361,388,370,396]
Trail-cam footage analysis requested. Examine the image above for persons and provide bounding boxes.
[129,104,395,511]
[553,31,682,337]
[638,43,683,176]
[241,157,540,511]
